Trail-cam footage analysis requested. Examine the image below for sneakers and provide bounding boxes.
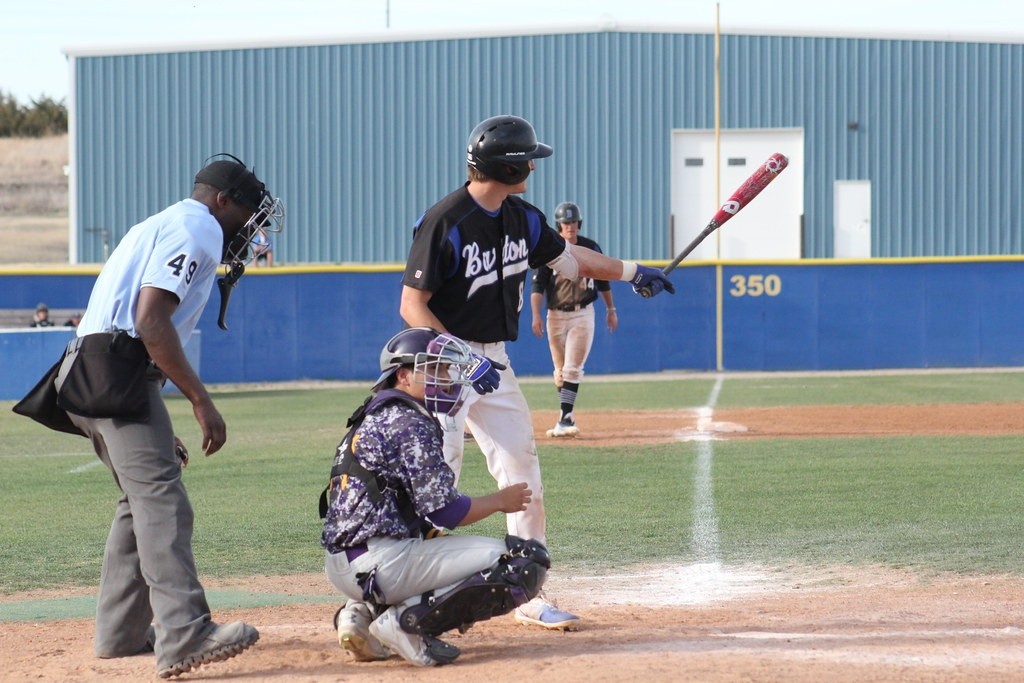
[158,622,258,678]
[137,623,157,653]
[546,422,579,436]
[338,607,392,661]
[514,595,579,630]
[369,606,439,666]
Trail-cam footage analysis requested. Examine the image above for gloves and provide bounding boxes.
[455,351,507,395]
[628,264,675,297]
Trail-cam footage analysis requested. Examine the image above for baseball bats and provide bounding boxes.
[640,152,788,298]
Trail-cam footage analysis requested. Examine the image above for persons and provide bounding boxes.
[30,302,55,327]
[531,201,618,436]
[252,227,273,268]
[399,115,677,626]
[63,311,81,326]
[54,153,285,675]
[319,327,551,668]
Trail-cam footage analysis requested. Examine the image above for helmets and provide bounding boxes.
[555,203,582,232]
[466,115,553,185]
[370,329,443,388]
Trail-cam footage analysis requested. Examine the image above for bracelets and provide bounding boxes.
[606,307,616,311]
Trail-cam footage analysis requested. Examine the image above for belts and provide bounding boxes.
[67,336,167,387]
[550,303,586,312]
[345,543,368,563]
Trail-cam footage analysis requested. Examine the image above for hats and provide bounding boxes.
[36,304,47,312]
[194,160,271,227]
